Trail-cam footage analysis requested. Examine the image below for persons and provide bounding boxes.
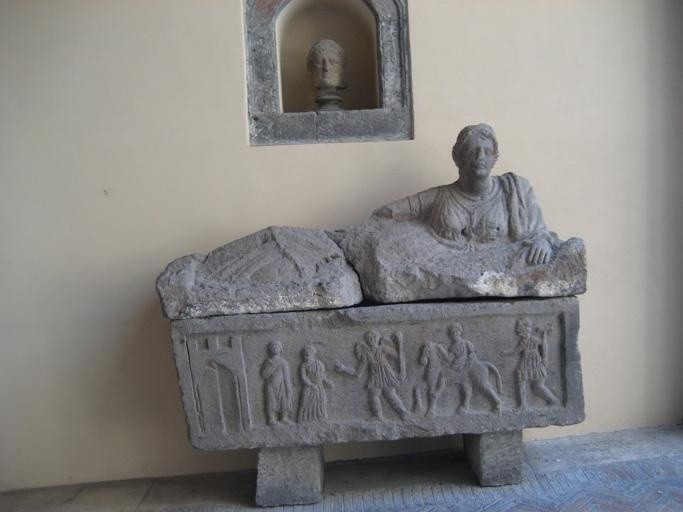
[333,329,412,421]
[260,339,294,427]
[296,343,335,423]
[429,323,477,401]
[306,40,342,90]
[384,123,561,265]
[500,317,559,411]
